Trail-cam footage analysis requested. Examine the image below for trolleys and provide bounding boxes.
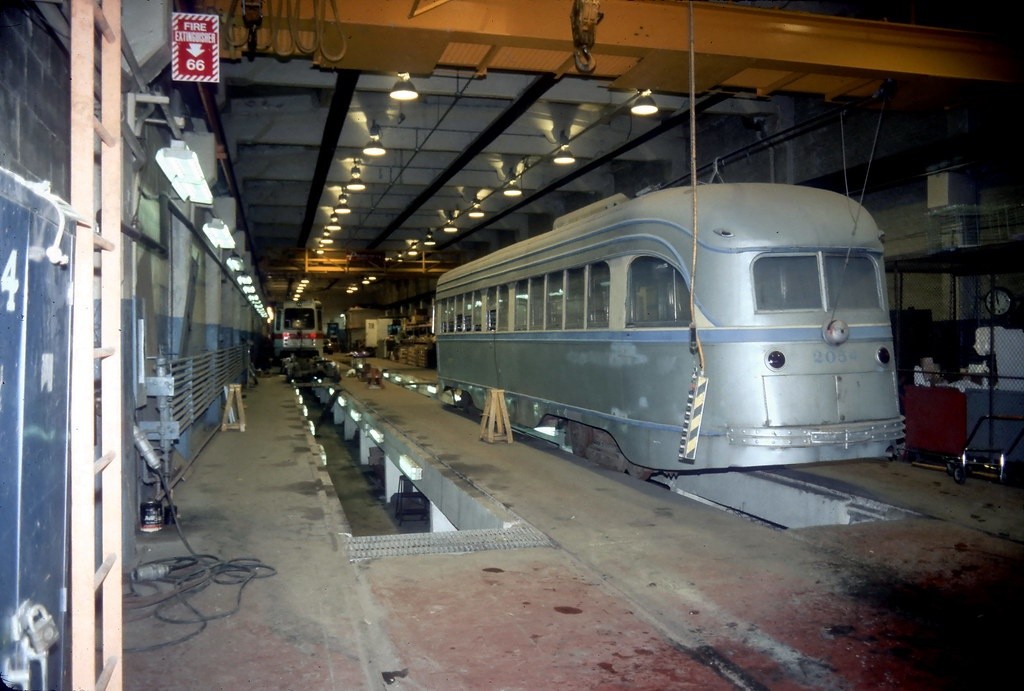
[885,414,1024,483]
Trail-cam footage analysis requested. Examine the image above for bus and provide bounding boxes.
[434,185,907,480]
[274,298,324,356]
